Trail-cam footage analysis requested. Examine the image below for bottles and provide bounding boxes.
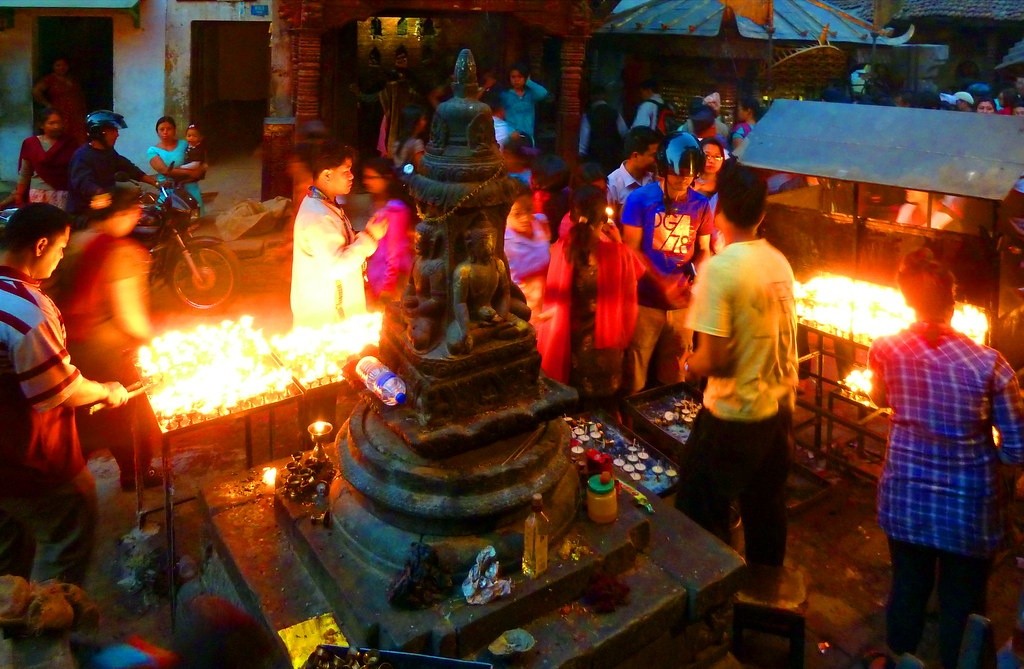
[570,445,618,523]
[522,494,548,581]
[355,355,407,407]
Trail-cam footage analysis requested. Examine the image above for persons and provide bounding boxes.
[0,58,1024,669]
[387,49,533,356]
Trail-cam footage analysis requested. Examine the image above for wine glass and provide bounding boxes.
[284,451,317,497]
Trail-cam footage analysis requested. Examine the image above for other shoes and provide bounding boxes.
[121,466,175,492]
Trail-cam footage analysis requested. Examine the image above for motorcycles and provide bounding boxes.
[0,160,242,317]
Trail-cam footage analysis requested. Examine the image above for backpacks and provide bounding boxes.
[633,98,678,136]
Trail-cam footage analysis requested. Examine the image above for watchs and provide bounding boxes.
[683,357,696,377]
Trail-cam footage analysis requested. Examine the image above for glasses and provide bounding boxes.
[706,155,723,160]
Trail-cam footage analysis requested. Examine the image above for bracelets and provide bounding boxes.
[156,182,159,186]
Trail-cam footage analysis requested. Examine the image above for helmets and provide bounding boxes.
[82,109,128,136]
[657,131,706,179]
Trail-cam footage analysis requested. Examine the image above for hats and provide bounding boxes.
[965,83,991,96]
[950,92,973,105]
